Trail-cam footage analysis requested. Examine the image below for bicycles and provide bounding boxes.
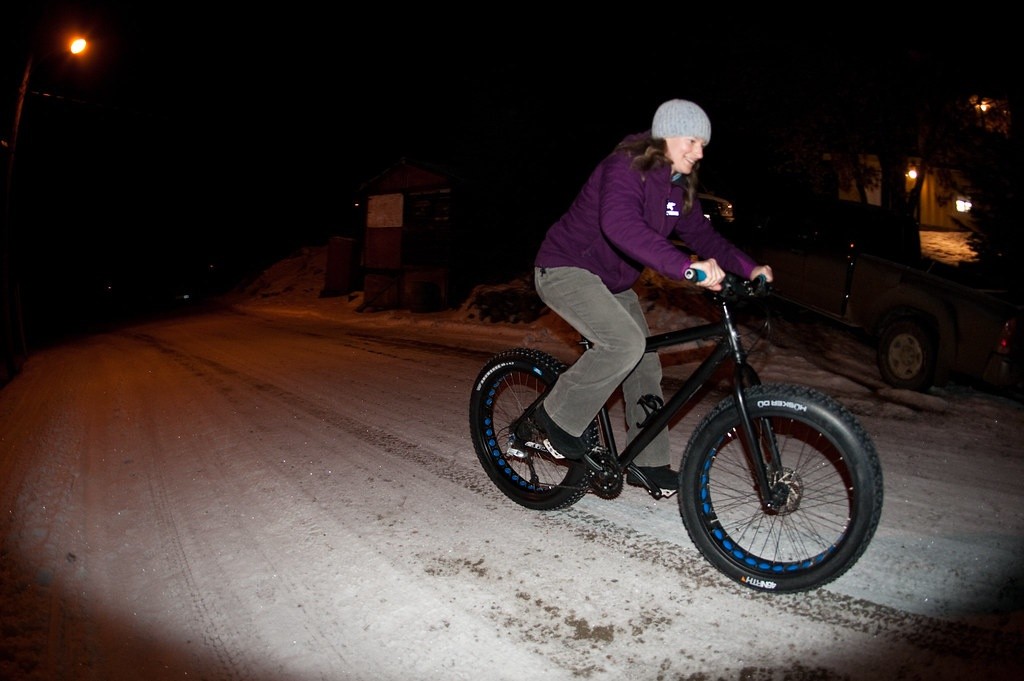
[470,269,880,590]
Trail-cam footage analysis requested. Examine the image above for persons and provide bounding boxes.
[510,99,774,492]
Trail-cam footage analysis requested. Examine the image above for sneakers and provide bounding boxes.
[627,463,680,490]
[532,402,586,460]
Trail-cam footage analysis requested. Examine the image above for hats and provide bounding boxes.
[652,99,712,147]
